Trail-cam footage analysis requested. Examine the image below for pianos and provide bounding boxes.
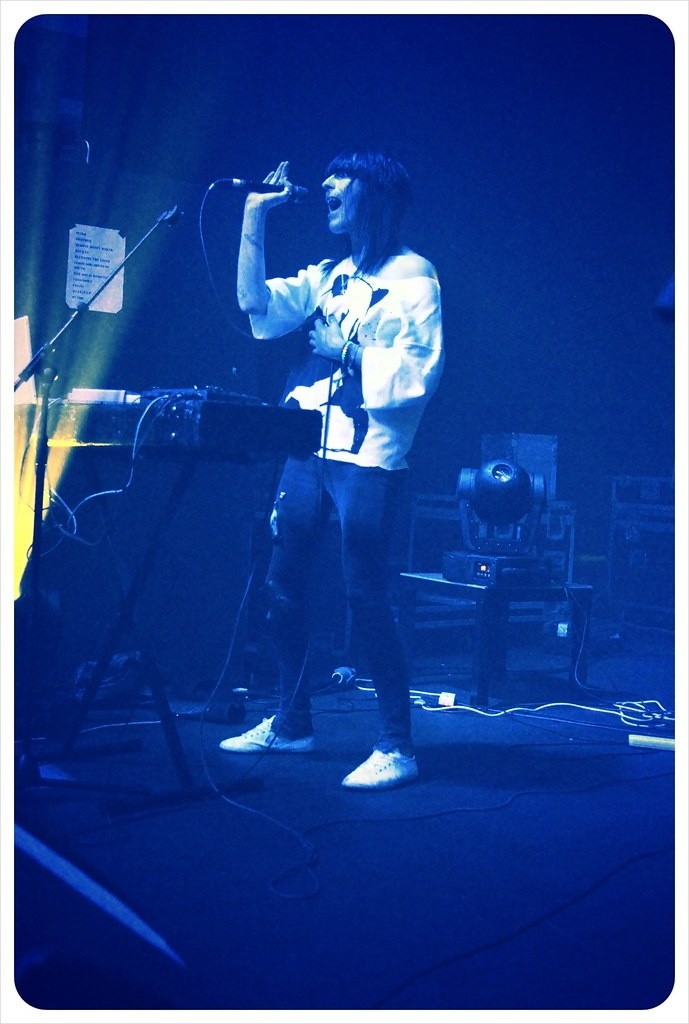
[29,385,325,462]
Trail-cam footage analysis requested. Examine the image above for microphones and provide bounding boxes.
[230,176,308,202]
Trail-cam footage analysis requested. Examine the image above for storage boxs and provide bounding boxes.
[409,494,577,624]
[609,473,675,515]
[609,508,675,635]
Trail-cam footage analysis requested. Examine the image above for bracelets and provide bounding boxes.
[241,229,259,239]
[340,341,359,370]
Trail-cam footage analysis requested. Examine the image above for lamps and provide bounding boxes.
[458,460,546,555]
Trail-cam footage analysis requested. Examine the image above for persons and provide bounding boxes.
[219,146,449,790]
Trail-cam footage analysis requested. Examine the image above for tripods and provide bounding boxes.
[14,367,202,816]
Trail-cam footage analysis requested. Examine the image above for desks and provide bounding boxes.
[400,572,595,704]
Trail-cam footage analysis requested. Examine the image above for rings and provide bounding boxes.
[285,187,293,193]
[329,319,337,323]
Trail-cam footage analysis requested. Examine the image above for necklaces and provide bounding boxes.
[342,255,353,294]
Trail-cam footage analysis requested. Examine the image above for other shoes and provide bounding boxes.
[219,715,316,753]
[341,750,419,790]
